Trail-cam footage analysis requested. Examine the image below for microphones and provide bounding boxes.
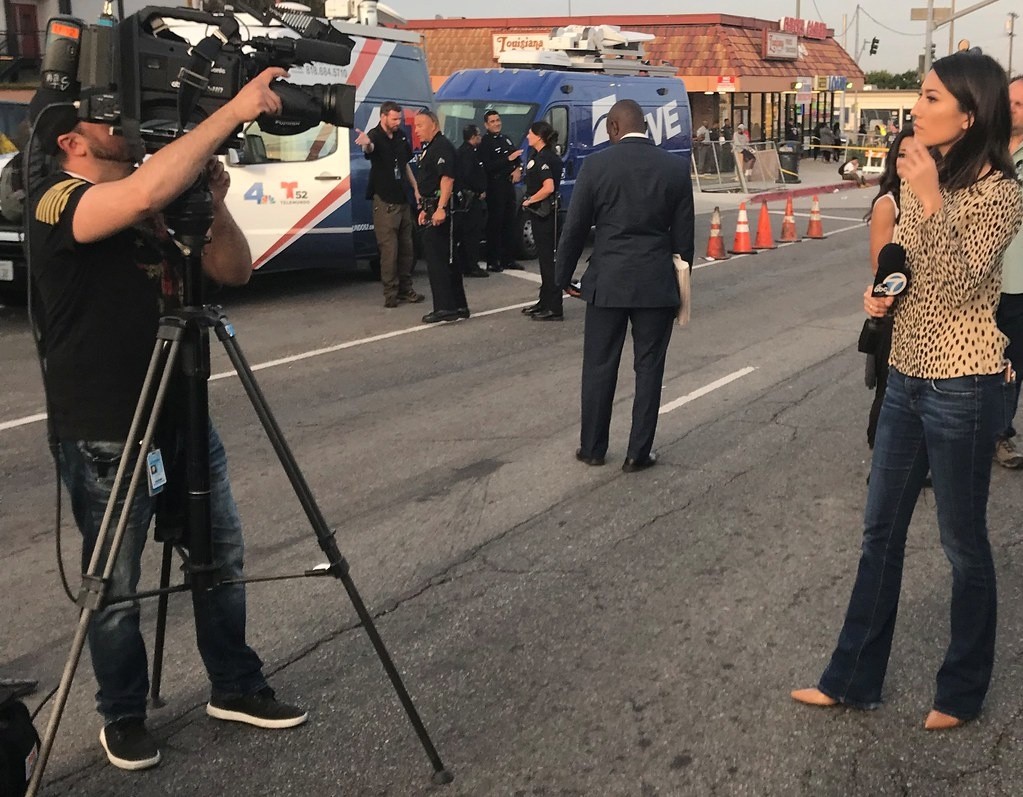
[858,243,906,356]
[269,37,352,66]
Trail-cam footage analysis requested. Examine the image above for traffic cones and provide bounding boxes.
[727,202,758,254]
[801,194,828,239]
[702,207,732,260]
[775,196,802,243]
[752,199,778,248]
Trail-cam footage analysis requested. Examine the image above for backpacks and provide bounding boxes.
[838,160,850,174]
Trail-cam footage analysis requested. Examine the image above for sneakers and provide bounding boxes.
[992,437,1023,467]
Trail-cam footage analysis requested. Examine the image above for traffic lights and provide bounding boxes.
[870,37,879,56]
[931,44,935,59]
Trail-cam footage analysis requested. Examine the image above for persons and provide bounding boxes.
[414,107,470,324]
[26,66,309,771]
[696,118,760,181]
[354,100,426,307]
[553,97,696,474]
[457,108,525,278]
[520,119,565,322]
[784,51,1023,730]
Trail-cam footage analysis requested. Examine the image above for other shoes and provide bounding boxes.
[397,287,426,304]
[464,266,489,278]
[521,302,564,321]
[486,263,503,272]
[744,168,752,175]
[622,454,656,473]
[421,306,470,323]
[575,447,605,467]
[791,687,837,706]
[924,710,960,729]
[502,261,525,270]
[99,717,161,769]
[859,182,872,187]
[383,296,399,308]
[206,686,309,729]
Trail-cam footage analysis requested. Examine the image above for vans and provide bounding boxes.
[0,0,437,283]
[433,24,692,259]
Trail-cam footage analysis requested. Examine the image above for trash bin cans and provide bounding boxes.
[693,141,734,174]
[775,142,800,184]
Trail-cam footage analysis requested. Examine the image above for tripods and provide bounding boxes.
[25,143,453,797]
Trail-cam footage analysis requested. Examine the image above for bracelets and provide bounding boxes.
[438,205,447,210]
